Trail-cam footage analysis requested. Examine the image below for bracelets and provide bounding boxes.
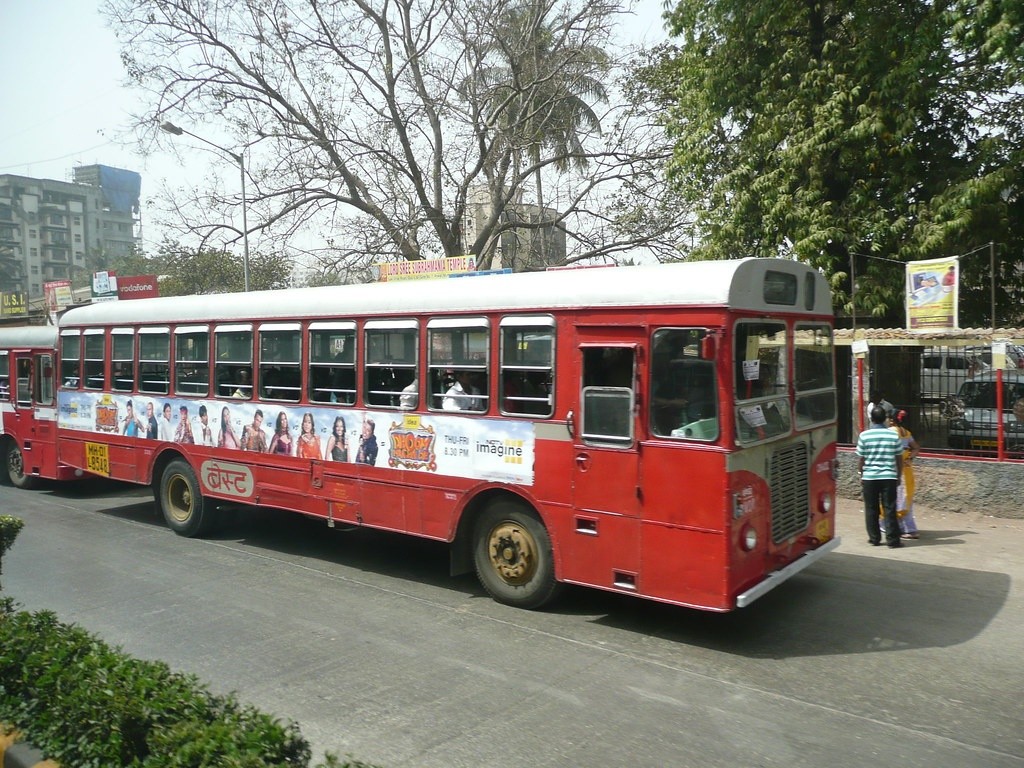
[858,472,862,475]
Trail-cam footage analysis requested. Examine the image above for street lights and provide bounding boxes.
[158,119,251,292]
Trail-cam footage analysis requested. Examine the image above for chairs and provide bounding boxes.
[216,369,236,396]
[180,374,209,393]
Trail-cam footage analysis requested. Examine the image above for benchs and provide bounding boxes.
[141,372,188,392]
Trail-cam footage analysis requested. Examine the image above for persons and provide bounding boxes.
[314,368,442,409]
[867,391,894,428]
[856,407,904,548]
[879,409,920,540]
[296,414,323,460]
[175,406,194,445]
[230,369,252,398]
[650,382,689,410]
[442,369,523,413]
[157,403,175,440]
[199,405,213,445]
[354,412,378,465]
[145,403,157,439]
[239,409,267,453]
[218,406,240,449]
[122,400,145,437]
[325,416,352,462]
[268,411,292,454]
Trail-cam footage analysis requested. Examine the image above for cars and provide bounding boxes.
[947,369,1024,460]
[920,339,1024,404]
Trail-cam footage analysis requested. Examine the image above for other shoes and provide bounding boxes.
[869,540,880,546]
[880,526,886,532]
[888,541,900,548]
[901,533,920,539]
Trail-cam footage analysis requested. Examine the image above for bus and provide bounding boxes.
[0,322,97,490]
[43,256,839,613]
